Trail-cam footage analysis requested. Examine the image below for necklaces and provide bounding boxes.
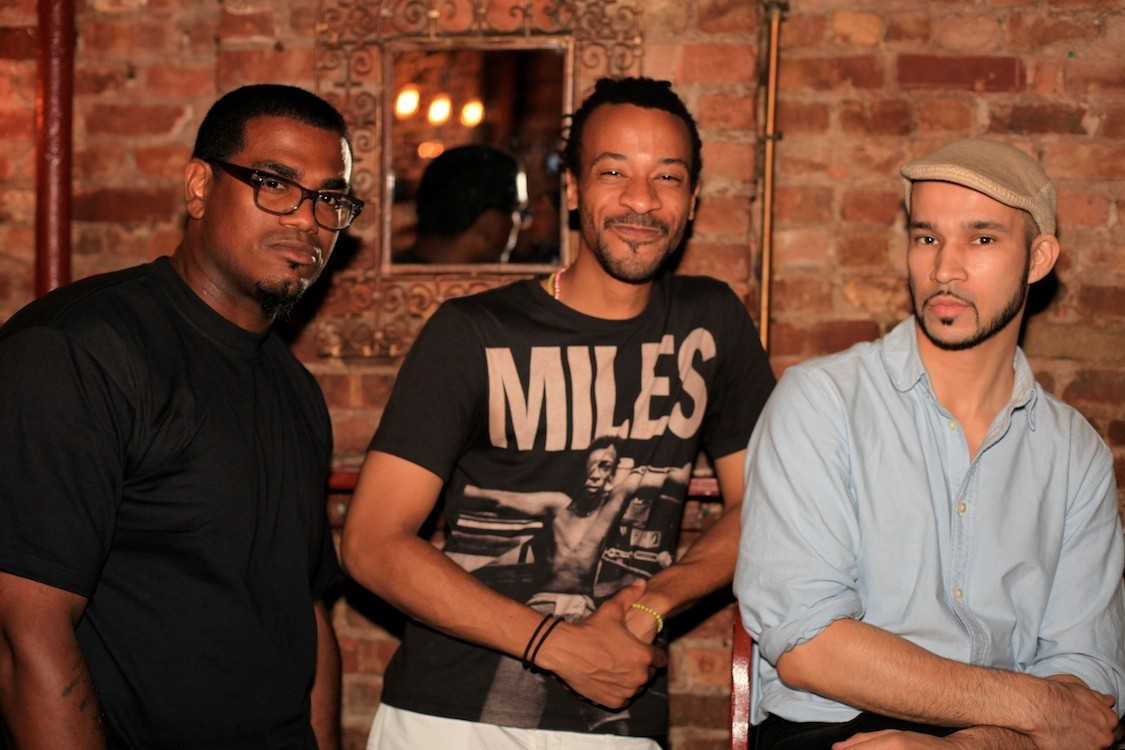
[547,266,568,303]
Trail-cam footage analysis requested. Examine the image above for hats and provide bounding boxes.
[899,137,1057,235]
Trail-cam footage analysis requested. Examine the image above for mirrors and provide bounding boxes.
[302,0,646,366]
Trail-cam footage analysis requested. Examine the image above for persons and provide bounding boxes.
[1,82,352,749]
[733,137,1124,749]
[340,77,778,750]
[393,144,528,265]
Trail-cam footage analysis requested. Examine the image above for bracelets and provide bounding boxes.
[522,614,564,667]
[631,601,663,633]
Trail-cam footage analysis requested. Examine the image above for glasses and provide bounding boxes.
[204,157,365,231]
